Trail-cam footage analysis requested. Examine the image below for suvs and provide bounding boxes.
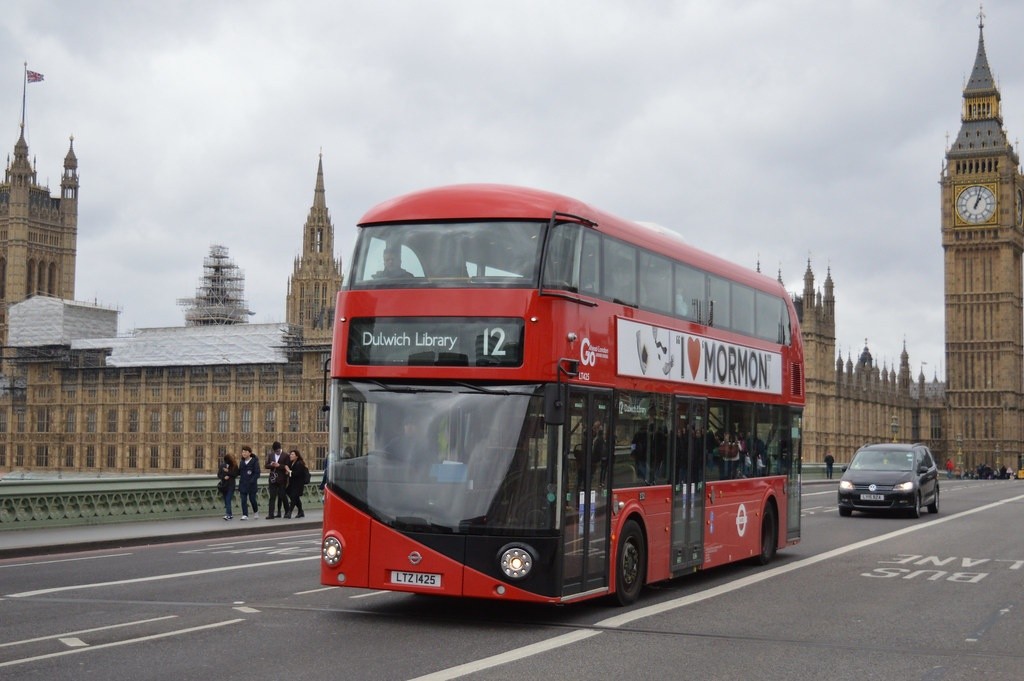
[838,443,941,519]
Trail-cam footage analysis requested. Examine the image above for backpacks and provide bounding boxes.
[297,460,310,485]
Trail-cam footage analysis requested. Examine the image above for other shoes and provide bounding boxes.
[254,511,258,519]
[240,515,248,520]
[284,511,291,519]
[295,510,304,518]
[266,515,274,519]
[223,514,233,521]
[274,514,281,518]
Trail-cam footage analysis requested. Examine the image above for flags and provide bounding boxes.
[27,70,44,84]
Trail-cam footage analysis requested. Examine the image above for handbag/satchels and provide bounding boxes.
[270,472,277,484]
[217,481,223,492]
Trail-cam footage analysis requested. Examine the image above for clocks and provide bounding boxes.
[954,184,995,226]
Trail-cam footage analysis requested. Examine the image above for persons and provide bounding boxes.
[945,459,953,478]
[217,453,239,521]
[343,446,353,458]
[577,423,770,487]
[824,451,834,479]
[266,440,305,519]
[238,446,261,520]
[963,462,1010,480]
[372,248,414,278]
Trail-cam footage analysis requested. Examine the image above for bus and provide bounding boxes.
[321,178,806,608]
